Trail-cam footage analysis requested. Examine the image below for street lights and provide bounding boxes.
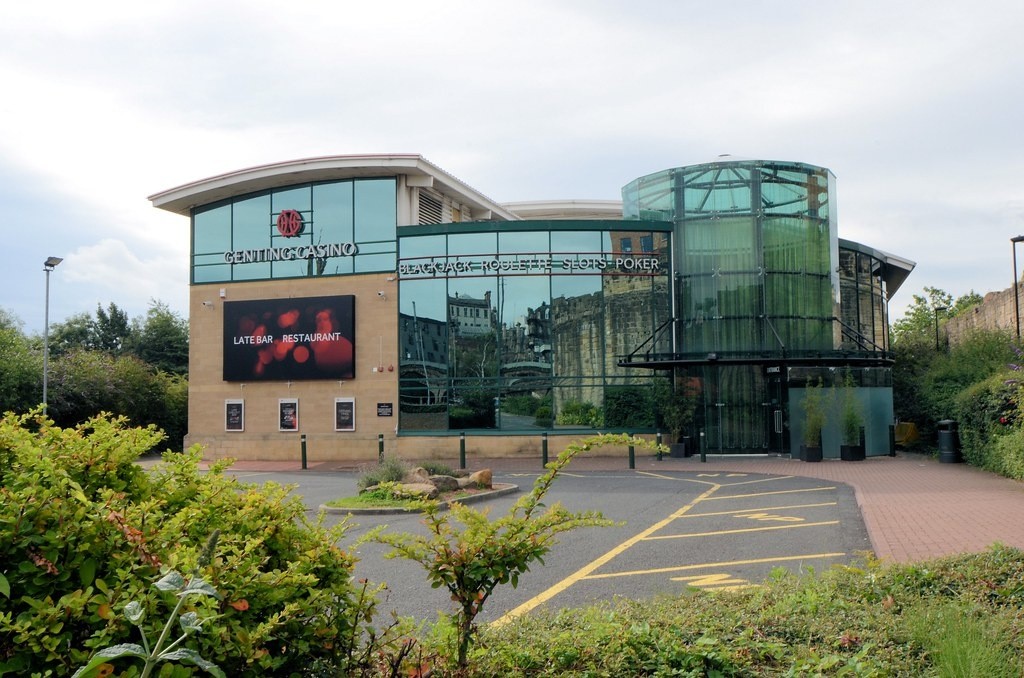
[40,256,63,416]
[934,306,947,350]
[1010,236,1024,339]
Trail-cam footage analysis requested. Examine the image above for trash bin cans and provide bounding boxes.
[937,419,958,463]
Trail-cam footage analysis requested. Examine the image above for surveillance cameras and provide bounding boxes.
[377,290,385,301]
[203,301,214,310]
